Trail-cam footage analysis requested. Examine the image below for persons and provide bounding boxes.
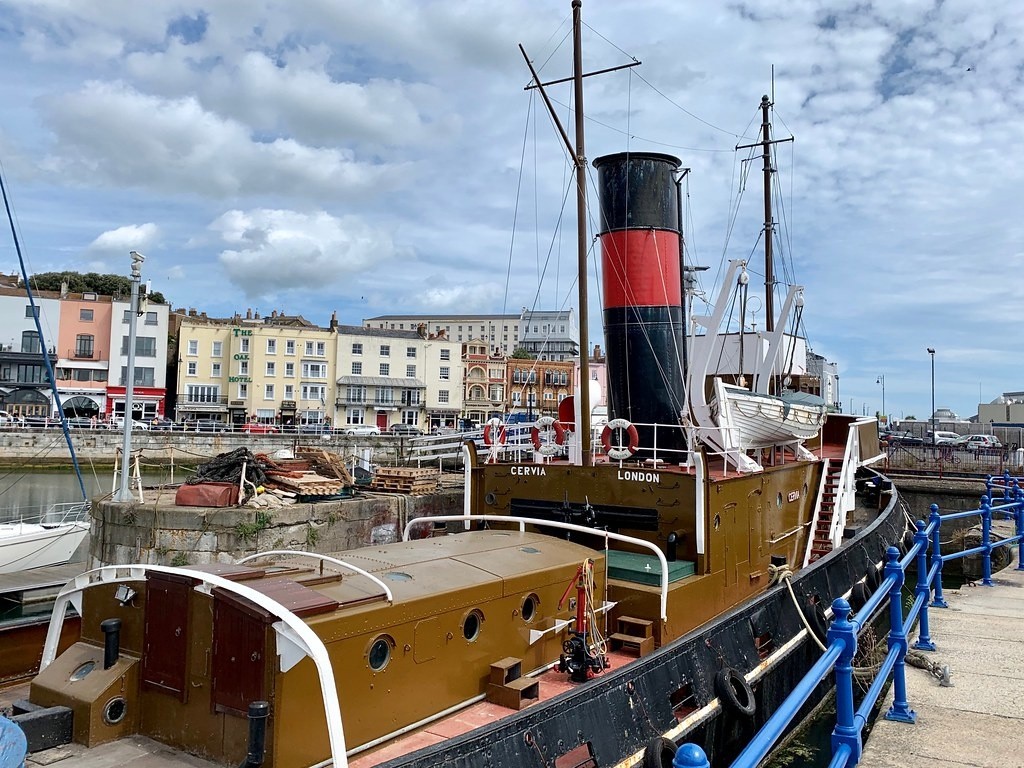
[323,420,330,433]
[152,418,159,429]
[181,415,188,431]
[46,416,60,423]
[92,415,97,429]
[286,418,292,425]
[8,413,28,428]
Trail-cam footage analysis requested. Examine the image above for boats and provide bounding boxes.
[0,0,935,768]
[706,377,829,449]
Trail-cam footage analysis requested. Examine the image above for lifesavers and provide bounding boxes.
[895,542,908,560]
[601,418,639,460]
[868,565,881,591]
[806,605,829,643]
[484,418,506,452]
[910,516,916,531]
[883,553,889,567]
[906,531,914,551]
[646,737,679,768]
[852,584,873,609]
[531,416,564,455]
[716,667,756,719]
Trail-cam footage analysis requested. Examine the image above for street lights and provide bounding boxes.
[876,374,884,416]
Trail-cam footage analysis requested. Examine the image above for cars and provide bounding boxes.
[188,418,232,433]
[879,428,973,448]
[956,434,1002,454]
[346,424,382,436]
[137,416,168,430]
[389,423,425,436]
[0,410,150,430]
[295,423,333,434]
[241,421,279,433]
